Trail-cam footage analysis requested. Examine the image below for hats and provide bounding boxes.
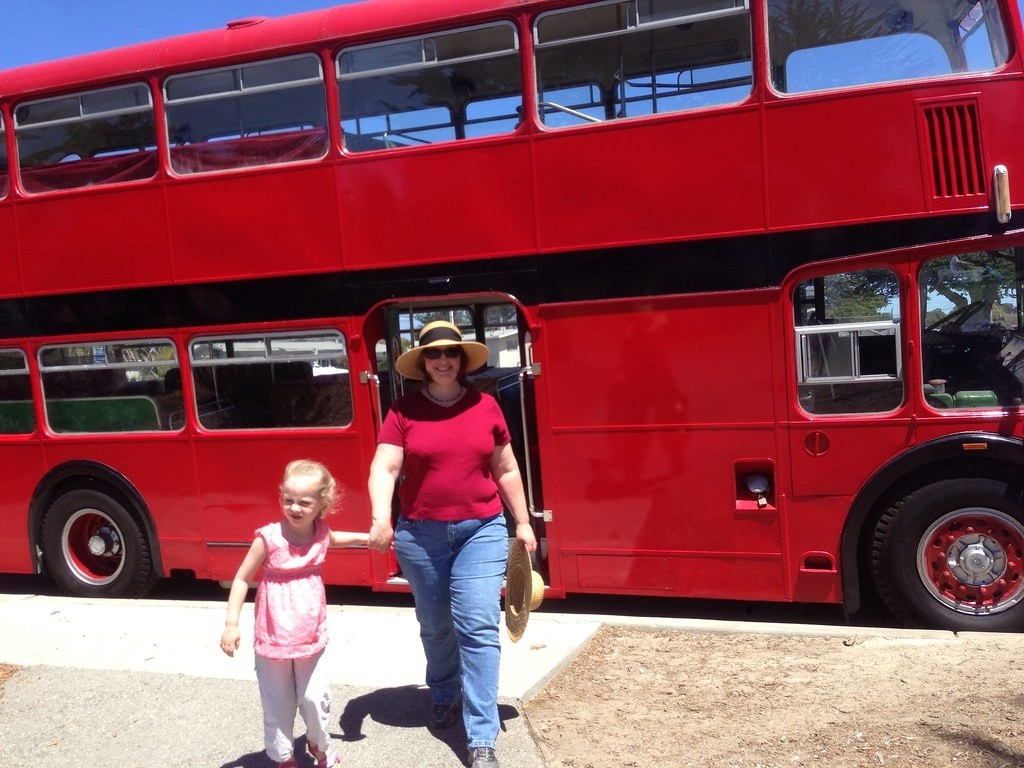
[395,320,489,380]
[504,541,544,642]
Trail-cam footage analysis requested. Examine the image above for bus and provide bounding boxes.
[0,0,1022,633]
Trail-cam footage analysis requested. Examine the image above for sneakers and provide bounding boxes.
[429,686,462,728]
[468,747,500,768]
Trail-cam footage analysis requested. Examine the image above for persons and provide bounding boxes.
[365,319,536,768]
[219,458,394,768]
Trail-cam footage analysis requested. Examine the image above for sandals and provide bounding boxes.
[308,738,341,768]
[274,753,299,768]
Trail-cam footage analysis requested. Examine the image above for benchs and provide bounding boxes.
[1,361,355,432]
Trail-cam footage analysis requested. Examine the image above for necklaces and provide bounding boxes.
[425,384,464,401]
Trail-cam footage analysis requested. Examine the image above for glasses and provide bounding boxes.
[423,347,461,359]
